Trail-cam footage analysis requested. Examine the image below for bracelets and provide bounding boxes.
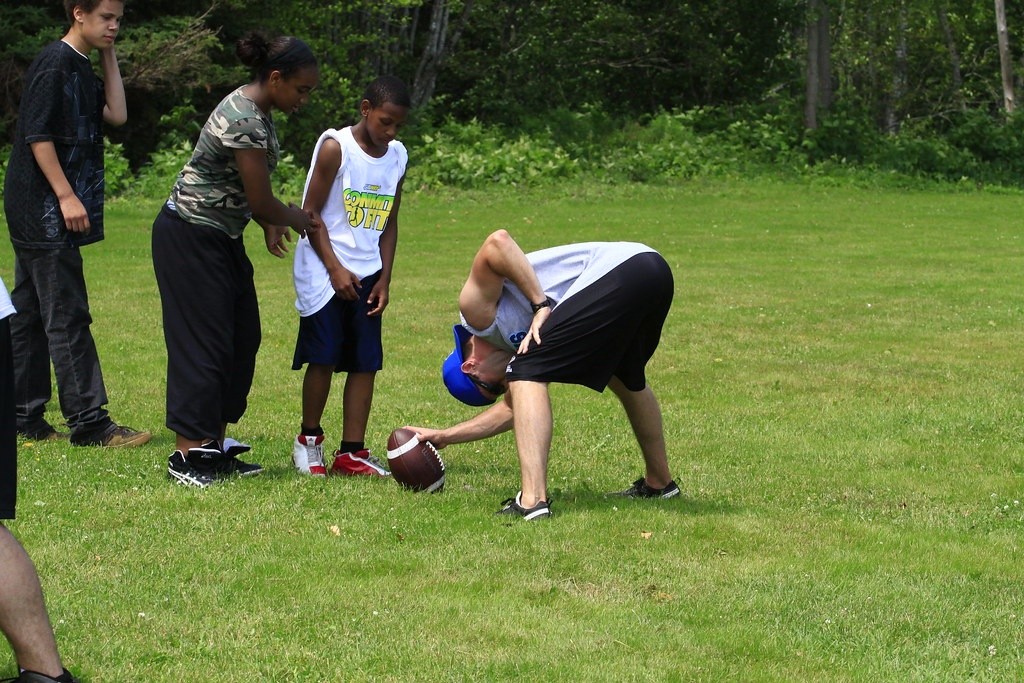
[528,299,551,312]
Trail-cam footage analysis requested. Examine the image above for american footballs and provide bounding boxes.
[387,428,446,495]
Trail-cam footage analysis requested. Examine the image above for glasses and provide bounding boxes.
[467,373,506,395]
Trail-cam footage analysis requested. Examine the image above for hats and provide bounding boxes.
[443,324,495,405]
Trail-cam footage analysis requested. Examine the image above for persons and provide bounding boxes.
[149,35,322,489]
[0,277,86,682]
[399,230,684,521]
[292,75,413,477]
[0,3,154,445]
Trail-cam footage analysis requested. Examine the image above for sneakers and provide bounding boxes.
[290,434,392,479]
[167,438,264,489]
[16,422,151,447]
[604,476,679,500]
[495,491,552,522]
